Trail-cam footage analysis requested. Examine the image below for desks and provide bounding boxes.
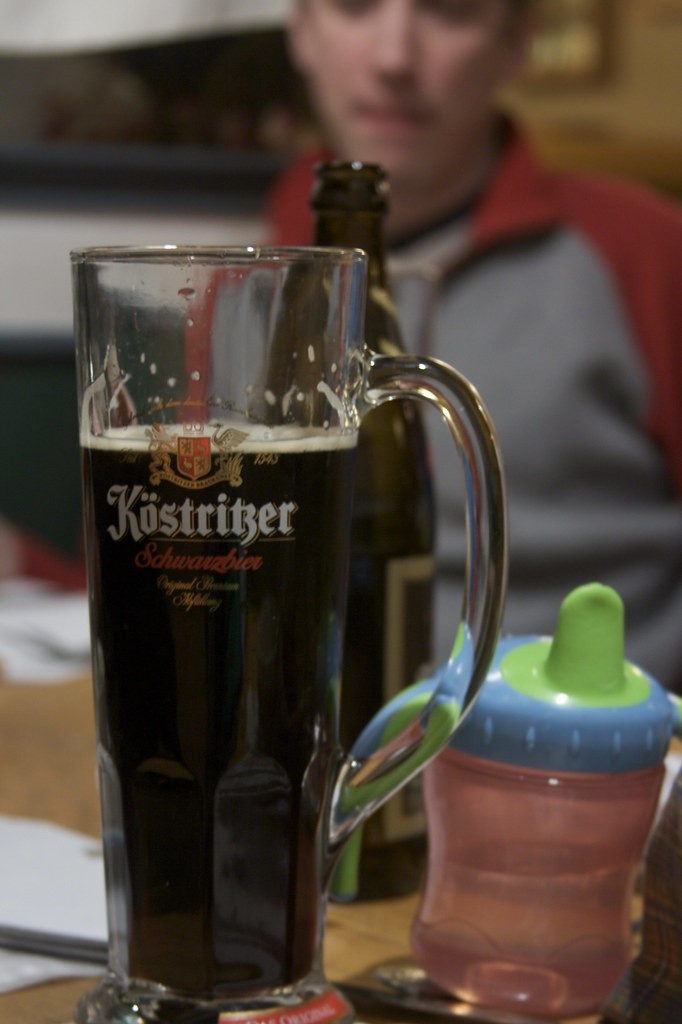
[0,606,682,1024]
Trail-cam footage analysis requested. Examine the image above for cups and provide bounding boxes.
[70,244,508,1024]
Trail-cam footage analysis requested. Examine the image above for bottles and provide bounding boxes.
[333,580,682,1019]
[253,161,439,906]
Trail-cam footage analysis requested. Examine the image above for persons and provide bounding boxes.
[2,3,681,691]
